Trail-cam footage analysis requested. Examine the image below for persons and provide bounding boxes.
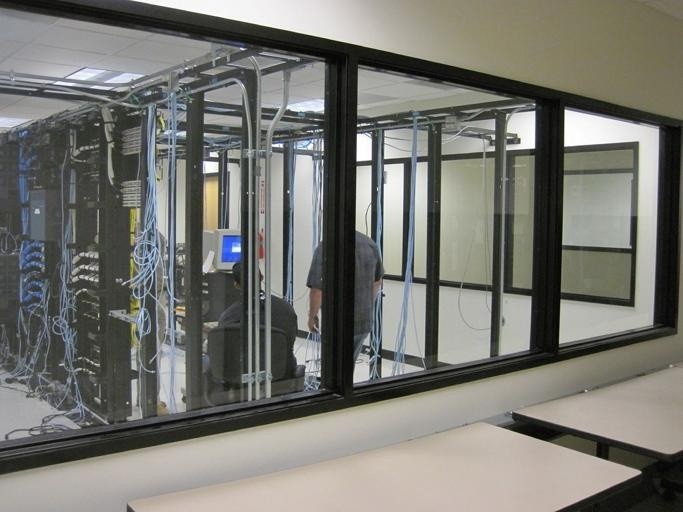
[217,261,297,378]
[306,229,386,374]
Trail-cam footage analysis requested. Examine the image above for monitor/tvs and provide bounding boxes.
[212,228,241,270]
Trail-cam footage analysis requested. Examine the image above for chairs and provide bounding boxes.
[203,324,304,408]
